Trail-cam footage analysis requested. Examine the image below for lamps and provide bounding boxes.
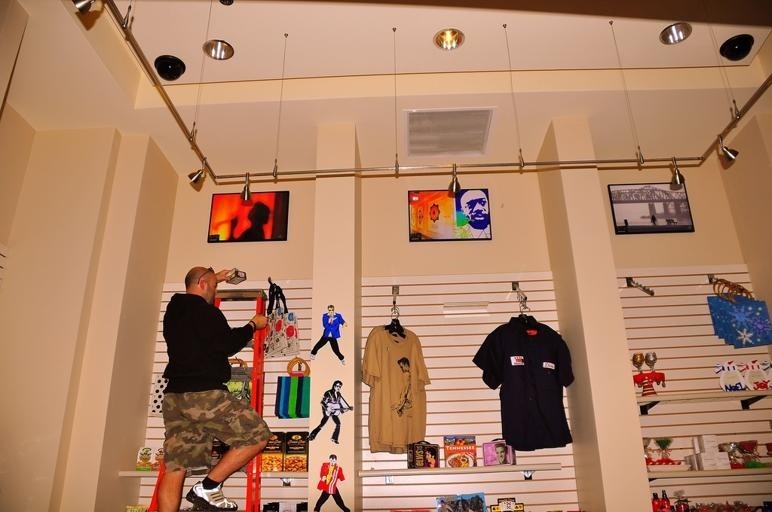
[710,132,739,168]
[241,156,691,201]
[74,0,207,186]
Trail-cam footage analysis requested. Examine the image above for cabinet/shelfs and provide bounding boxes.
[637,390,772,480]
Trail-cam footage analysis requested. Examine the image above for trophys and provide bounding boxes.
[717,441,741,469]
[736,439,763,468]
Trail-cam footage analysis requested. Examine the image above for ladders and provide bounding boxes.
[147,289,268,512]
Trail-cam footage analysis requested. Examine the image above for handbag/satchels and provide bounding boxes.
[274,376,310,419]
[707,293,772,349]
[261,307,299,358]
[223,359,265,410]
[150,376,170,414]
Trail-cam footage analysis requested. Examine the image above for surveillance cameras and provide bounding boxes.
[154,54,186,82]
[720,34,754,62]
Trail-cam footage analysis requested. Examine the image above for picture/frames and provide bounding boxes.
[407,188,492,242]
[207,191,289,243]
[608,182,695,235]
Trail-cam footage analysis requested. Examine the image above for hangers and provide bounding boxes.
[508,291,541,336]
[385,306,407,339]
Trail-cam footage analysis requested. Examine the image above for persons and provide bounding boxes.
[494,444,509,463]
[650,214,657,225]
[310,304,349,366]
[312,454,351,511]
[423,446,438,467]
[228,201,270,241]
[460,190,491,240]
[438,498,484,512]
[395,357,416,416]
[308,381,352,443]
[159,266,269,512]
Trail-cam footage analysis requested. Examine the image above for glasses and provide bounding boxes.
[197,266,213,284]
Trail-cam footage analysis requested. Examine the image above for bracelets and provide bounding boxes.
[249,320,257,332]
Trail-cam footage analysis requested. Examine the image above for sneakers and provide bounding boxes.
[185,481,238,512]
